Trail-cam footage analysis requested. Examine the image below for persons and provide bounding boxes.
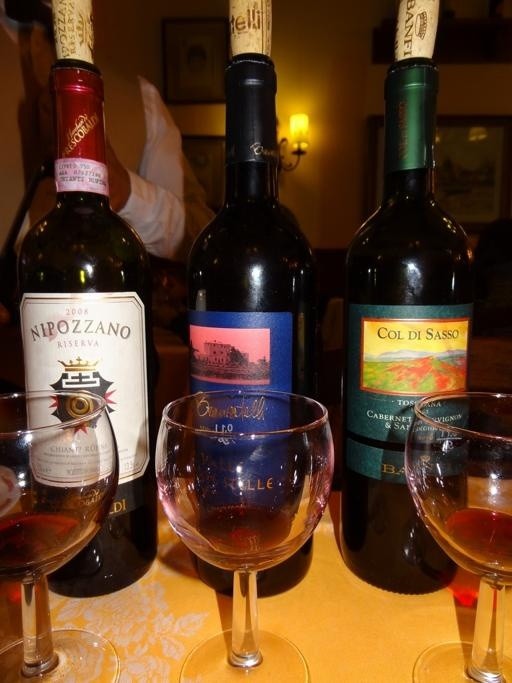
[0,1,217,327]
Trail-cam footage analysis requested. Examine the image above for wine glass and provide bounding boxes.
[404,391,512,683]
[0,389,120,683]
[155,389,335,683]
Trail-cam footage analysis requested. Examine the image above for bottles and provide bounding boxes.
[184,0,318,598]
[17,0,159,598]
[341,0,477,595]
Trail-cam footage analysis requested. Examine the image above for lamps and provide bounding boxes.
[278,113,311,171]
